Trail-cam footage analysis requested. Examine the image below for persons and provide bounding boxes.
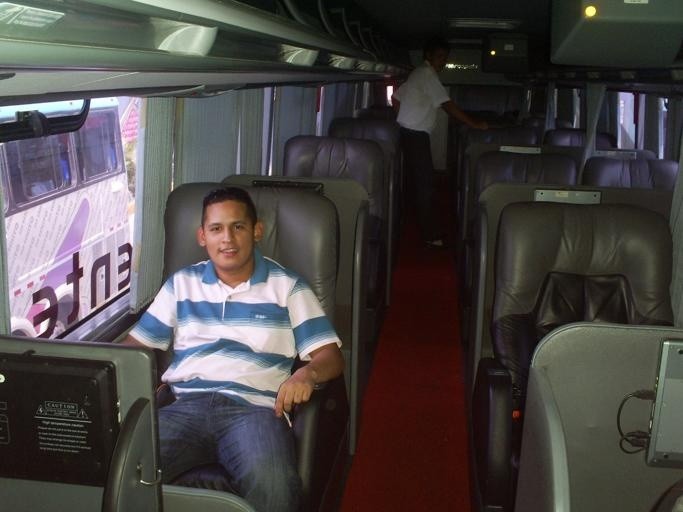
[391,34,490,250]
[116,188,347,512]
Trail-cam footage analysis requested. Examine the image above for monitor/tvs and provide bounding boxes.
[499,145,541,154]
[252,179,324,196]
[642,337,683,470]
[0,335,164,512]
[597,150,637,160]
[534,189,602,204]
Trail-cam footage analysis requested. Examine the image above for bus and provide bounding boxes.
[0,96,135,344]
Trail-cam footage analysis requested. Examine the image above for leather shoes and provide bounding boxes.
[423,238,451,250]
